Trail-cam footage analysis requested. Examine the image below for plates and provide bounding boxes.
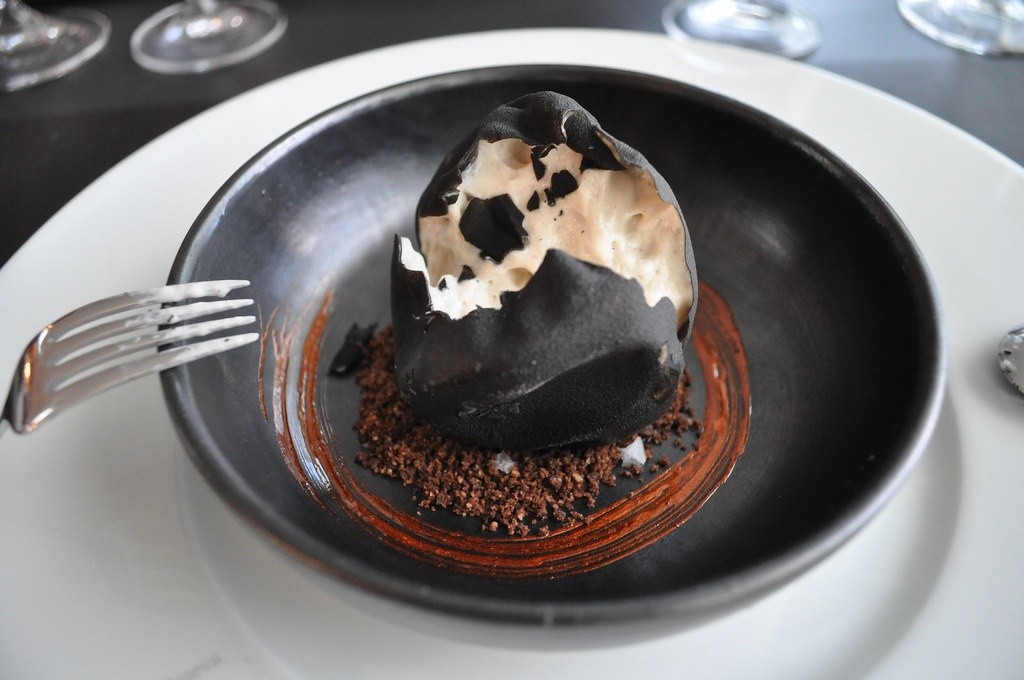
[0,28,1024,679]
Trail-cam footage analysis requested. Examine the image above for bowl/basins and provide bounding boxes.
[160,61,943,626]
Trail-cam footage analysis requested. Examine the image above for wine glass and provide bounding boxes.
[129,0,289,75]
[0,0,112,94]
[654,0,1024,63]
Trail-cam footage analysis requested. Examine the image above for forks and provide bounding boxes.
[0,280,259,439]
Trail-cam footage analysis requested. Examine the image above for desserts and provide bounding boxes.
[389,90,698,452]
